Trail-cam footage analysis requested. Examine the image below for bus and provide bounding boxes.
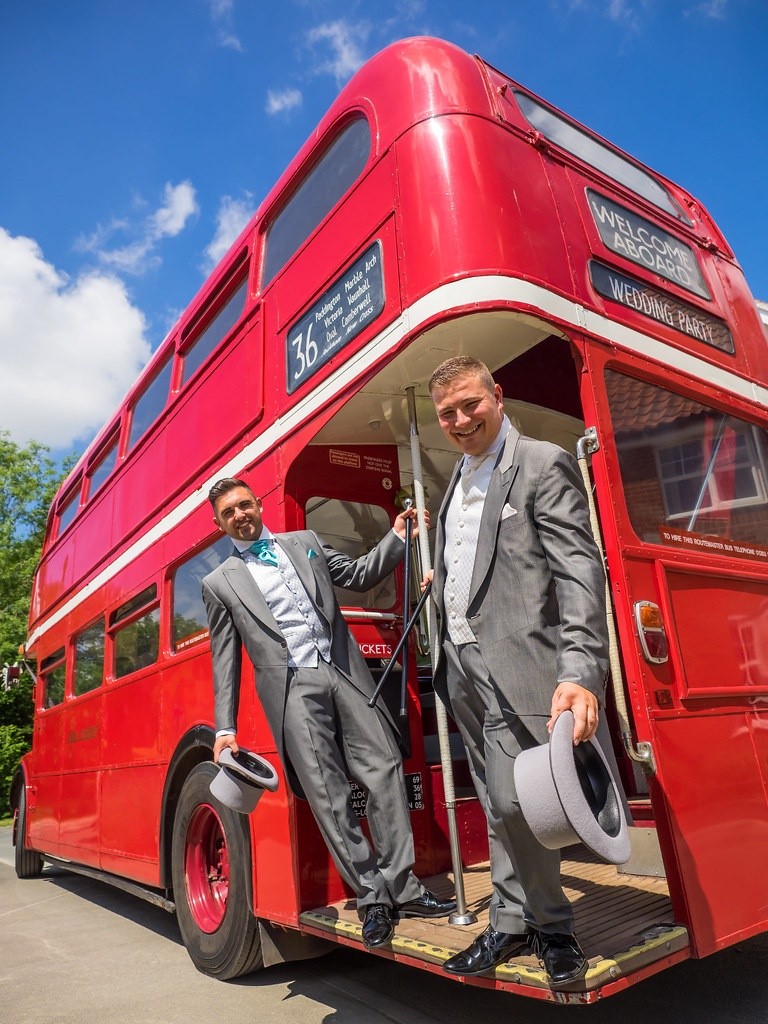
[10,35,768,1010]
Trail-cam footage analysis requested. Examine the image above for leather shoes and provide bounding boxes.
[530,930,588,988]
[362,903,393,951]
[391,890,457,919]
[442,923,534,977]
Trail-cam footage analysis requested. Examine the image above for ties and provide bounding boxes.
[249,539,279,568]
[460,454,486,476]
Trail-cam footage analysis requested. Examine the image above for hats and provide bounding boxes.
[210,746,278,815]
[514,710,632,865]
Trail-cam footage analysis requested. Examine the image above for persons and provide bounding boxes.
[196,476,461,948]
[426,357,608,987]
